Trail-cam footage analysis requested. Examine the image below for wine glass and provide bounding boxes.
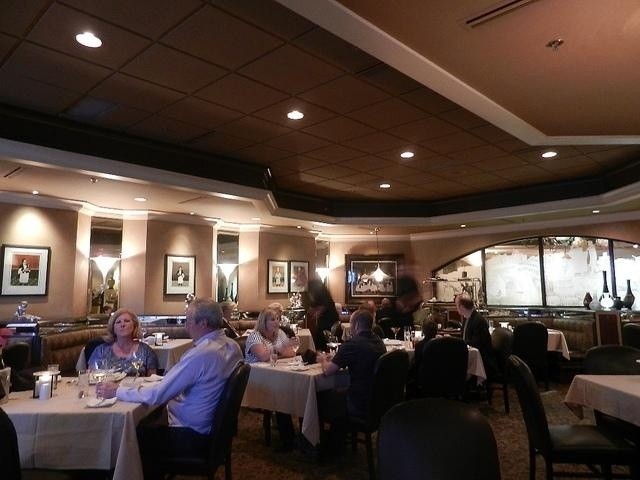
[78,357,144,399]
[391,326,411,349]
[269,340,301,369]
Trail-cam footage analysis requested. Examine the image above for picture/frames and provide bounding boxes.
[345,254,404,304]
[290,261,309,293]
[164,255,196,295]
[268,259,290,294]
[0,244,51,296]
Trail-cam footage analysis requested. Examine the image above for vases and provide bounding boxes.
[584,271,635,310]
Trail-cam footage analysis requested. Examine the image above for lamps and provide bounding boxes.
[368,227,394,282]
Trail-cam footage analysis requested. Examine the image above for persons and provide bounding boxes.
[247,268,493,465]
[96,297,244,480]
[176,265,185,286]
[88,308,158,378]
[220,302,239,338]
[16,258,30,285]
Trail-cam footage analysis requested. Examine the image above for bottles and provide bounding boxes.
[598,270,635,310]
